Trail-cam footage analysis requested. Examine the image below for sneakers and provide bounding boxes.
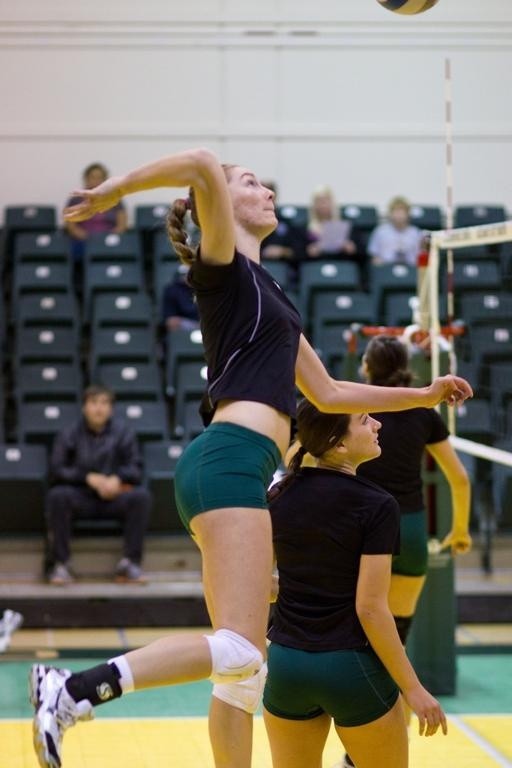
[47,561,73,584]
[27,663,95,767]
[117,562,147,582]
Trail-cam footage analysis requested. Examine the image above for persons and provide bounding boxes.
[64,163,127,260]
[44,382,152,584]
[29,147,475,768]
[158,181,427,338]
[340,335,472,768]
[264,399,447,768]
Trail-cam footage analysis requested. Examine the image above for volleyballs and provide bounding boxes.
[377,0,438,16]
